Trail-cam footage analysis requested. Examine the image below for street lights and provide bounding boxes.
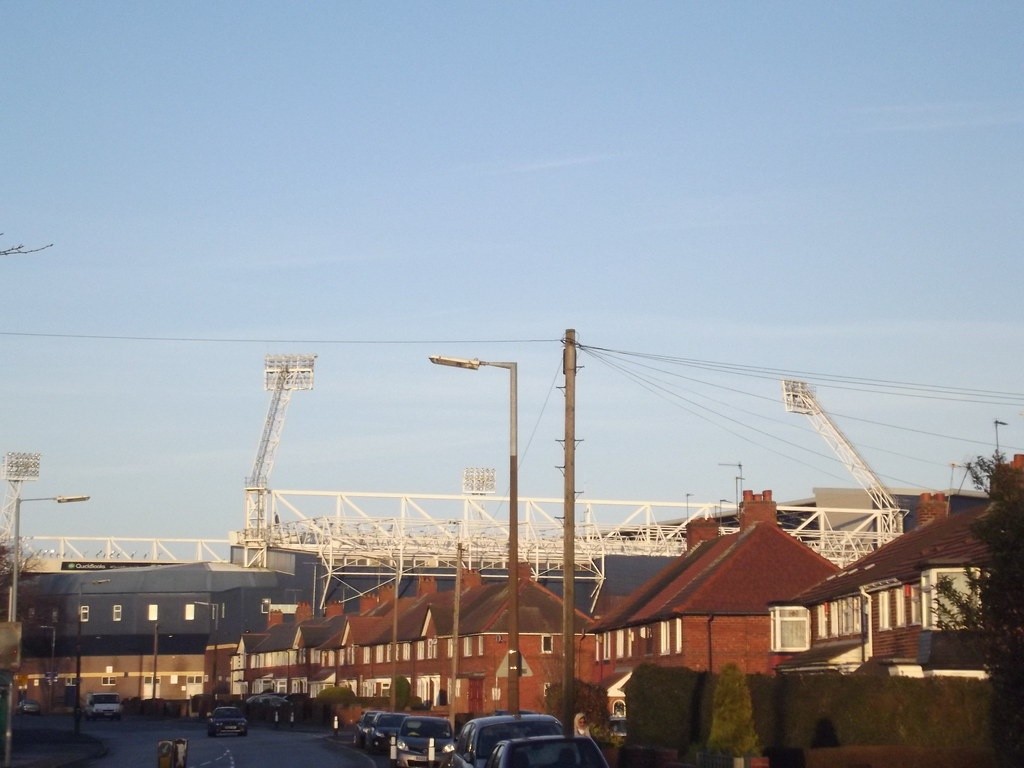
[994,420,1009,452]
[4,495,91,768]
[429,355,520,715]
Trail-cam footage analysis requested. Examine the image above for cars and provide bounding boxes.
[389,714,458,768]
[451,711,611,768]
[206,707,249,737]
[352,710,387,749]
[18,698,40,715]
[362,712,410,756]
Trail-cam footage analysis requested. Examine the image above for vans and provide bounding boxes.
[83,691,124,721]
